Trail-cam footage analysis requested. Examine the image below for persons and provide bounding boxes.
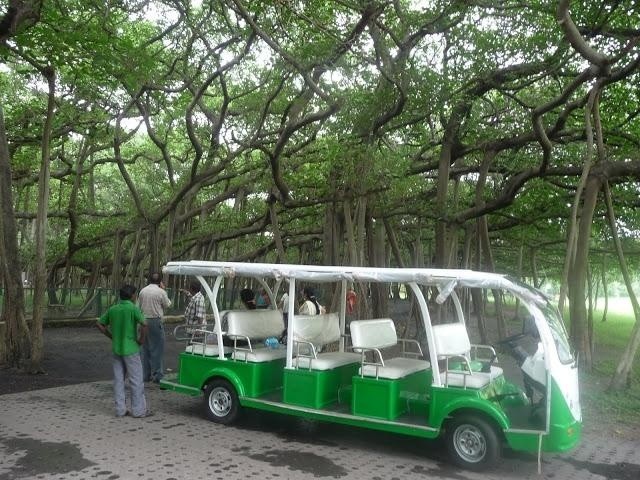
[140,271,173,385]
[346,284,358,316]
[257,287,271,308]
[277,292,289,344]
[207,288,256,347]
[95,285,156,418]
[298,285,326,353]
[180,278,208,348]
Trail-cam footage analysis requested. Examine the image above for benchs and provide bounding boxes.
[350,318,431,380]
[432,323,504,388]
[185,309,287,363]
[293,313,362,370]
